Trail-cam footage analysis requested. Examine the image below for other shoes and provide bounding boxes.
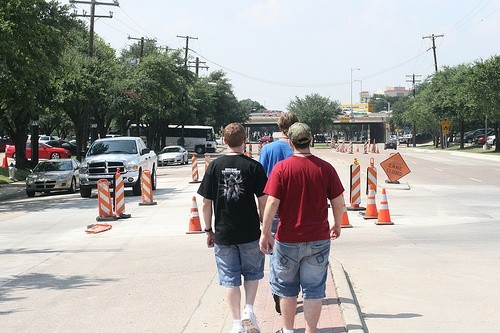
[233,321,244,333]
[273,294,281,315]
[241,308,261,333]
[275,328,283,333]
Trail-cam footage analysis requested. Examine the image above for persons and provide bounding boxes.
[258,122,345,333]
[260,113,300,313]
[197,121,268,333]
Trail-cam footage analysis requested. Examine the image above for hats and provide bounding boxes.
[287,122,311,144]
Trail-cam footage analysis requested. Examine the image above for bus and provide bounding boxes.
[128,123,217,155]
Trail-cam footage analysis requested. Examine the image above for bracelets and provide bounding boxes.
[205,228,212,232]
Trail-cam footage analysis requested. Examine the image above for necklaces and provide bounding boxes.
[279,137,288,139]
[228,152,241,153]
[293,152,311,154]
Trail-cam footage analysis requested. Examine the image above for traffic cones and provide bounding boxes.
[341,192,351,229]
[185,196,205,234]
[1,153,8,169]
[334,138,380,154]
[363,185,379,219]
[375,188,395,225]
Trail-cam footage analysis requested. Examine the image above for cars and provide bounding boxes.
[25,159,81,197]
[5,141,71,160]
[158,145,190,166]
[77,135,158,197]
[339,108,367,115]
[384,139,396,150]
[27,135,91,155]
[457,128,500,144]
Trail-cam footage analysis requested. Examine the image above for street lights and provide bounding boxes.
[376,97,392,137]
[350,66,360,117]
